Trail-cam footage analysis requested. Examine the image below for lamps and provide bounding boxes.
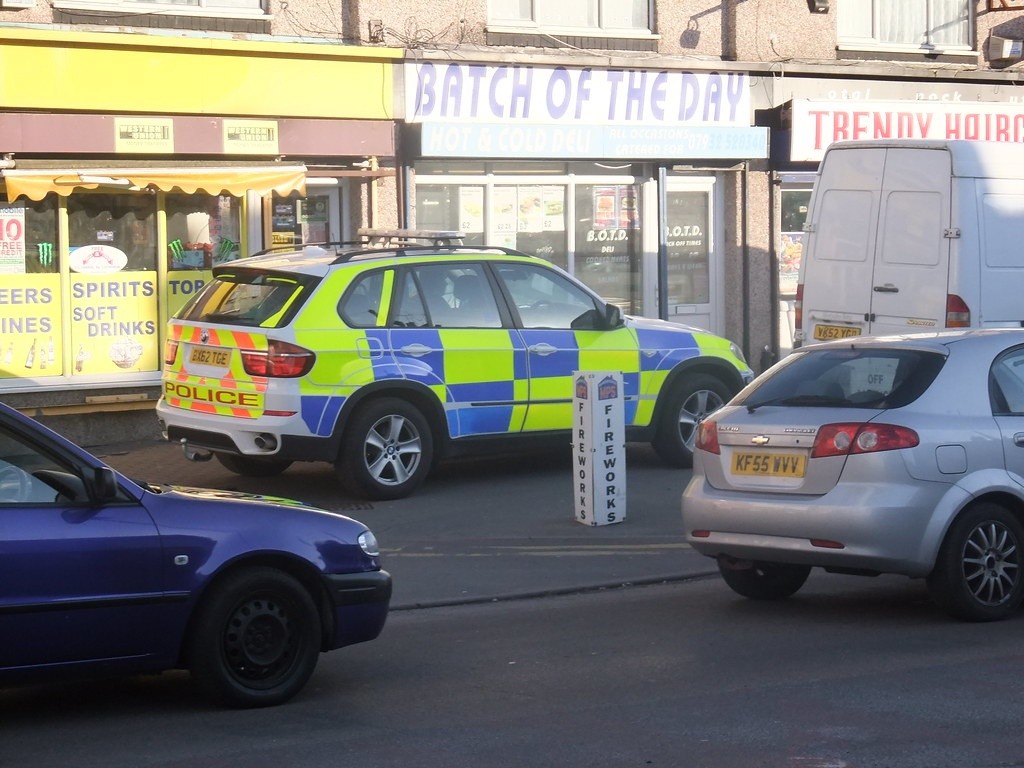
[806,0,828,14]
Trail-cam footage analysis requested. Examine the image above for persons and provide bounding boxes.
[407,265,451,310]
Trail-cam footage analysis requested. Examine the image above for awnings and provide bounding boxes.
[2,165,307,204]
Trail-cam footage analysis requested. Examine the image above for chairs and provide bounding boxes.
[452,275,489,325]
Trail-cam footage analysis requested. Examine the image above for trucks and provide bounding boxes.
[791,138,1024,353]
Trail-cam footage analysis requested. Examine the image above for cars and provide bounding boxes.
[154,226,755,503]
[679,327,1024,624]
[0,400,394,709]
